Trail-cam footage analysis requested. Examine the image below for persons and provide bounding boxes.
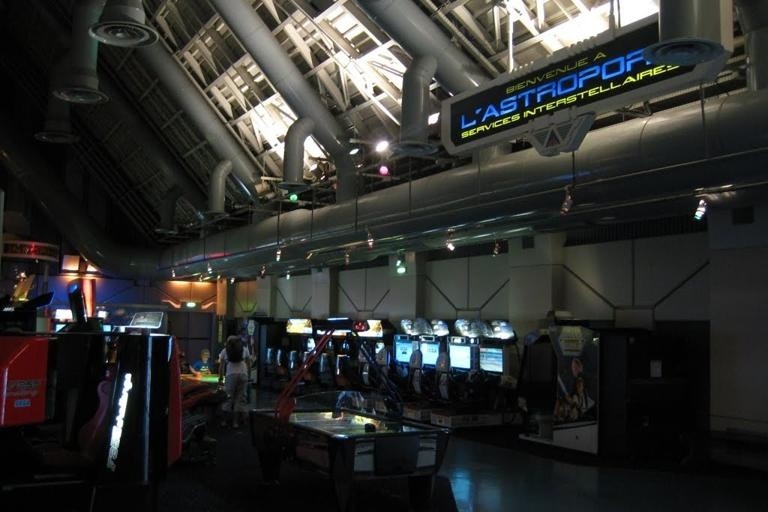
[188,348,215,377]
[217,335,252,387]
[388,360,405,394]
[217,344,249,428]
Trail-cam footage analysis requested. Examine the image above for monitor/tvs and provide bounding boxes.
[269,334,521,379]
[52,304,167,334]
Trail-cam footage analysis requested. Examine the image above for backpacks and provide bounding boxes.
[226,339,244,362]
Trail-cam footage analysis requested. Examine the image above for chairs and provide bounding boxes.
[262,344,490,408]
